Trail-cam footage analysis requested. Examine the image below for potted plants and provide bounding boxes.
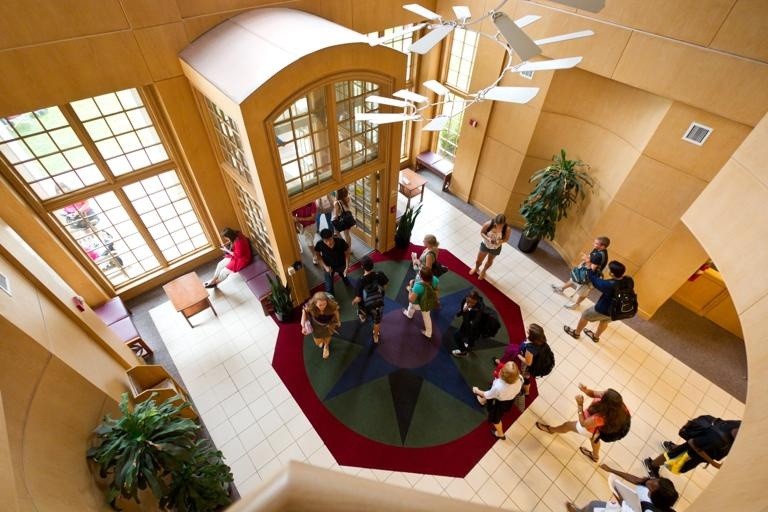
[395,203,422,249]
[517,148,597,253]
[264,270,294,321]
[87,391,235,511]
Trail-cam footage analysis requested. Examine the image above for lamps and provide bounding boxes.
[353,0,596,133]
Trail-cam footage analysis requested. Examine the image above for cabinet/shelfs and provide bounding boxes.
[125,365,198,421]
[670,261,744,345]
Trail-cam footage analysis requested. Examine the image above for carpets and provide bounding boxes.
[270,242,538,479]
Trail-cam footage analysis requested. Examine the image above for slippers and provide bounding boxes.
[372,330,379,343]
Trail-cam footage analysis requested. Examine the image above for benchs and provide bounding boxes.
[417,151,454,191]
[239,245,282,316]
[92,296,153,355]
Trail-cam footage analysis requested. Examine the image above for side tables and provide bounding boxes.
[162,271,218,329]
[398,167,429,211]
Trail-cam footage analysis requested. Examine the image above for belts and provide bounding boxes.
[311,317,333,326]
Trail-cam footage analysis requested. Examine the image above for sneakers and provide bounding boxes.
[551,284,562,293]
[662,440,675,450]
[562,302,579,310]
[452,349,468,357]
[644,457,659,477]
[566,503,577,512]
[313,257,318,265]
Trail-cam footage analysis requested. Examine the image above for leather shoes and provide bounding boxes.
[205,282,217,288]
[493,356,500,365]
[420,328,429,338]
[489,429,506,440]
[402,308,412,319]
[205,283,208,285]
[319,343,323,348]
[474,394,485,407]
[469,268,476,275]
[477,273,484,280]
[322,347,329,359]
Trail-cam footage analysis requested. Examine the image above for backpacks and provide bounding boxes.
[678,415,723,442]
[528,341,554,377]
[611,278,638,322]
[419,283,438,312]
[593,412,631,444]
[360,275,384,310]
[570,266,590,285]
[432,262,448,278]
[478,307,500,339]
[320,196,332,213]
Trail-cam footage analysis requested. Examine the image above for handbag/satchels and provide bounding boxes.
[300,304,313,335]
[331,200,356,232]
[515,376,525,412]
[425,251,448,278]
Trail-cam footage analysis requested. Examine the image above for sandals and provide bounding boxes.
[536,422,553,434]
[583,328,600,342]
[580,446,599,462]
[563,325,580,340]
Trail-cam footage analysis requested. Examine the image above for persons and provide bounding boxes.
[402,267,440,338]
[565,464,679,512]
[551,236,611,310]
[316,198,334,233]
[493,323,546,397]
[535,383,623,463]
[301,293,340,358]
[314,228,351,297]
[472,361,525,440]
[643,420,741,476]
[352,255,390,344]
[333,188,352,255]
[406,234,438,289]
[204,228,253,288]
[563,259,626,343]
[292,203,318,264]
[452,290,486,357]
[468,214,511,280]
